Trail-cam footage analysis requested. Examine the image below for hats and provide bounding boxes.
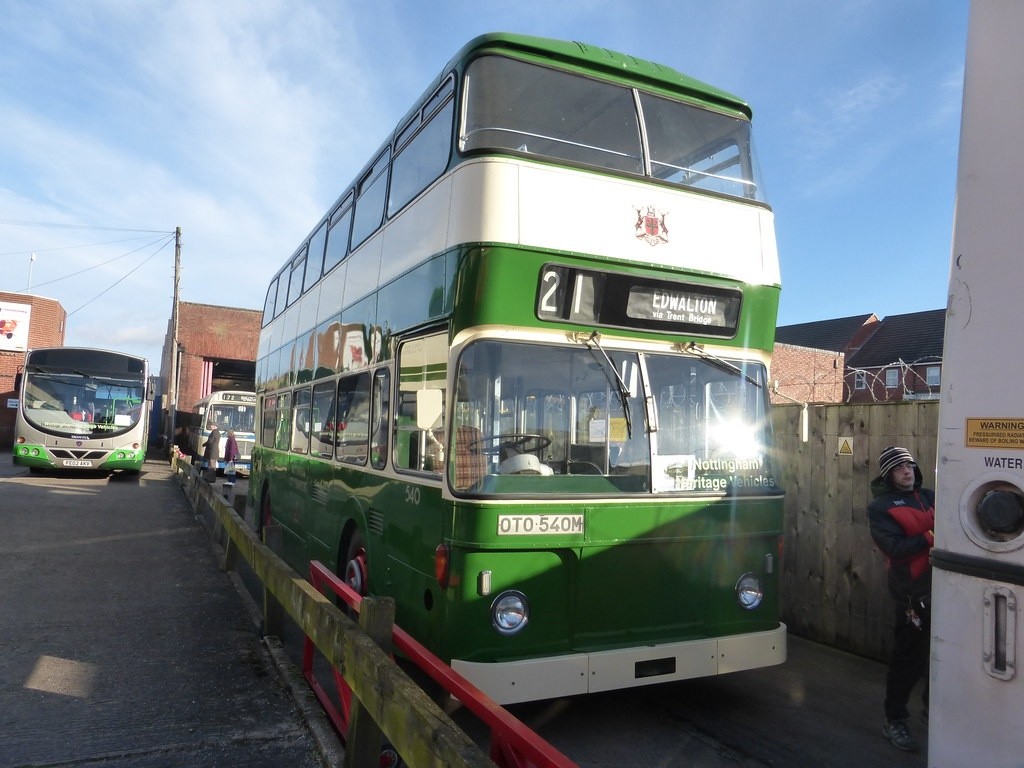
[877,446,914,477]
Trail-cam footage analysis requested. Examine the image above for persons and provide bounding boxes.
[866,446,936,750]
[222,430,238,486]
[202,422,221,483]
[328,416,346,447]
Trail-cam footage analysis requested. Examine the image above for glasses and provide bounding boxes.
[892,463,917,472]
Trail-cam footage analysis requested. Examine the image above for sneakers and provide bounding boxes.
[882,717,918,752]
[918,706,929,724]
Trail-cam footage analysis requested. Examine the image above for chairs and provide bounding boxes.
[428,424,487,493]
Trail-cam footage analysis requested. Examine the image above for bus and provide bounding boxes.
[186,390,257,478]
[249,31,808,708]
[261,404,321,454]
[12,347,155,477]
[310,390,380,464]
